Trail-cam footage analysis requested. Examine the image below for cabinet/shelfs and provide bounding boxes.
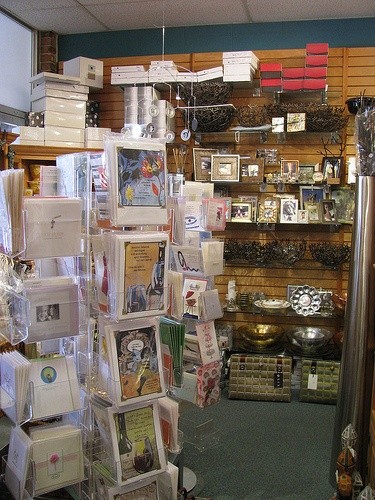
[79,77,355,394]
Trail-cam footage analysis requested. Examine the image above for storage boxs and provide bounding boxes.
[11,56,198,148]
[305,42,329,56]
[222,51,260,65]
[223,58,259,70]
[197,66,224,83]
[282,67,305,79]
[222,74,253,82]
[260,77,282,93]
[305,54,328,68]
[223,64,256,79]
[303,78,326,90]
[282,79,304,91]
[260,63,282,78]
[304,66,328,78]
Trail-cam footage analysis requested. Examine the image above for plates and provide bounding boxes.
[254,299,291,313]
[289,285,322,317]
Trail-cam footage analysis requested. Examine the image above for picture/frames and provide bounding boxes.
[259,205,278,222]
[231,201,252,222]
[298,210,309,223]
[280,159,300,180]
[192,148,219,182]
[328,184,356,224]
[304,201,322,223]
[299,165,315,181]
[280,199,298,223]
[299,185,327,211]
[321,157,344,183]
[262,197,280,222]
[210,154,240,182]
[240,158,265,183]
[318,199,337,224]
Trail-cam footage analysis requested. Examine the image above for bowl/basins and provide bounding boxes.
[285,326,334,353]
[237,323,283,348]
